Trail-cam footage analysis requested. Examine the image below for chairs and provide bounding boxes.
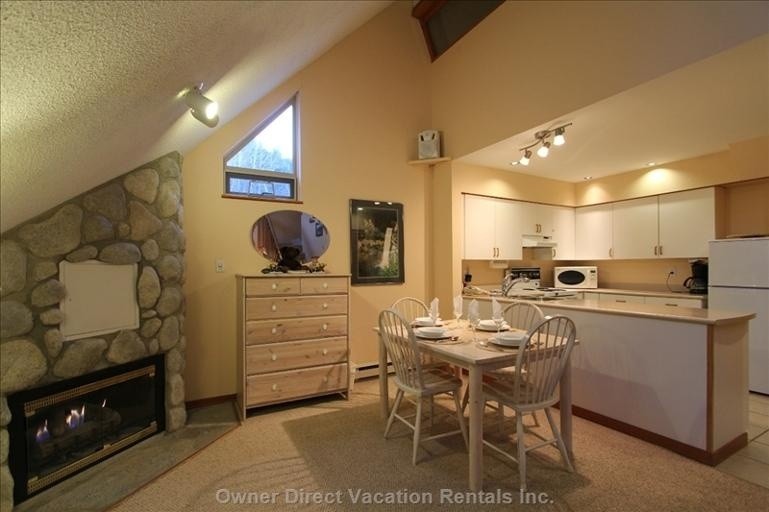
[458,300,546,433]
[379,309,473,465]
[480,313,579,495]
[381,296,465,435]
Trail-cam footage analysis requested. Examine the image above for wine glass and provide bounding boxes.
[468,313,482,348]
[493,312,505,334]
[453,307,464,329]
[428,305,440,326]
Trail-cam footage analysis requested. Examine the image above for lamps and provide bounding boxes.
[518,123,572,166]
[186,85,219,129]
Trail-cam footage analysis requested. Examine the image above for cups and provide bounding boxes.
[463,274,472,287]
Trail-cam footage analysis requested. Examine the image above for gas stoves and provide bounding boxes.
[510,280,577,297]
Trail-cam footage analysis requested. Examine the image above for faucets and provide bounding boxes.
[501,272,530,297]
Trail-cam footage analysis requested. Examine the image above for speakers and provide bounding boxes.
[417,128,441,159]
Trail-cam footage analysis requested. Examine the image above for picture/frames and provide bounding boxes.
[348,197,405,286]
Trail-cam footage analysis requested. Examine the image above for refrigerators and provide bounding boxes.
[708,237,769,396]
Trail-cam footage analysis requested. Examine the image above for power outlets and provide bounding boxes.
[668,266,676,276]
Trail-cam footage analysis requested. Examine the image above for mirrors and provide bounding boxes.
[250,208,333,272]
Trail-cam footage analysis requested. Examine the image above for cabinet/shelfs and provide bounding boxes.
[463,192,523,261]
[574,200,612,262]
[598,291,645,307]
[235,272,352,426]
[553,205,575,261]
[611,185,728,261]
[523,202,553,238]
[644,295,708,309]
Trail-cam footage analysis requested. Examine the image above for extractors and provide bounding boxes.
[521,235,557,248]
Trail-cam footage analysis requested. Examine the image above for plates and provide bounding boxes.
[410,317,454,339]
[476,320,535,347]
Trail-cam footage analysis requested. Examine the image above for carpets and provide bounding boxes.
[281,393,594,512]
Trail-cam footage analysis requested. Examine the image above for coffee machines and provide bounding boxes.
[683,262,708,294]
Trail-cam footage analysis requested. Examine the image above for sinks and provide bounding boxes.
[509,295,563,301]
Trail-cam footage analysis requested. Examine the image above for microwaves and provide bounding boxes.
[553,266,598,289]
